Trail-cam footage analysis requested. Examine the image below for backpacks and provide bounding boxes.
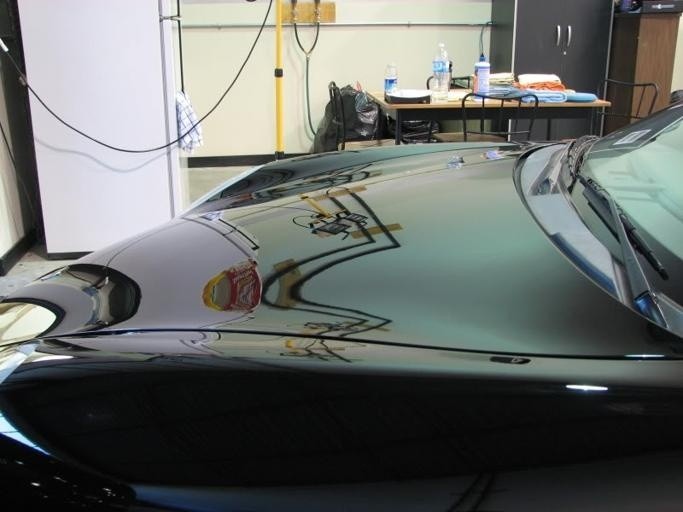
[314,81,385,152]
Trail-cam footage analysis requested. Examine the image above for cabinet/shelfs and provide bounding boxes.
[490,0,616,143]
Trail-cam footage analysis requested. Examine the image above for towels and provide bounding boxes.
[177,90,203,150]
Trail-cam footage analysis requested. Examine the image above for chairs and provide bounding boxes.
[328,81,405,152]
[426,75,474,133]
[432,93,539,142]
[594,79,658,141]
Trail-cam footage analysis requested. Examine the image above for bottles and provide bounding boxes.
[383,63,397,98]
[430,42,449,104]
[473,51,489,101]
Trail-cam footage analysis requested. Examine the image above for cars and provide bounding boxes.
[0,102,682,511]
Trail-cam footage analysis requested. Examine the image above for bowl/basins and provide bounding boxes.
[386,88,430,102]
